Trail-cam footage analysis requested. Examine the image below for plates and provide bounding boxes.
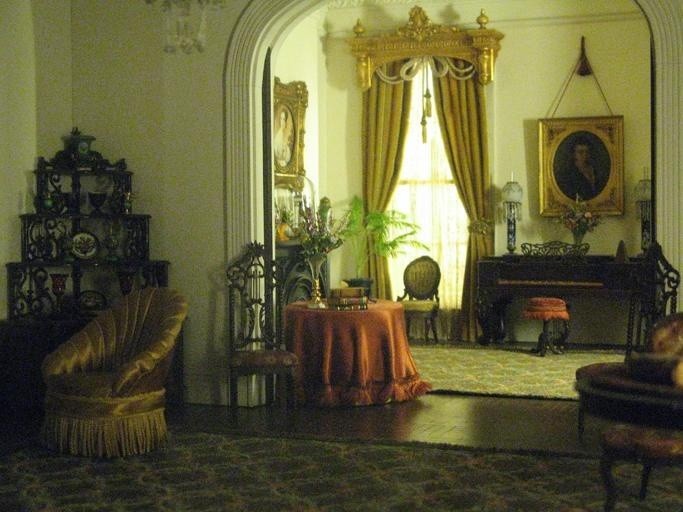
[73,290,107,312]
[67,230,101,262]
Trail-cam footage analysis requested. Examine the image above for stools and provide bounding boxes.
[519,298,570,355]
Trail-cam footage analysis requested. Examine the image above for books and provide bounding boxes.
[327,286,370,312]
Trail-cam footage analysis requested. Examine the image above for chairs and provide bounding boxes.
[598,423,683,512]
[40,288,189,458]
[396,256,441,344]
[624,311,683,361]
[225,240,298,424]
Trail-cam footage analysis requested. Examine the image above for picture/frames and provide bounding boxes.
[273,76,308,191]
[537,116,624,218]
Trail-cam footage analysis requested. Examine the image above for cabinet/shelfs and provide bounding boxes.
[9,129,168,315]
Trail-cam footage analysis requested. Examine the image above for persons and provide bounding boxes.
[273,107,292,168]
[556,136,602,202]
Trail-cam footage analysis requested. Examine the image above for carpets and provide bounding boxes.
[0,430,683,512]
[406,336,625,401]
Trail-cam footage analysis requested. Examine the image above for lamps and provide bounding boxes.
[632,166,652,250]
[500,172,523,253]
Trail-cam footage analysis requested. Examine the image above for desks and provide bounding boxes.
[476,253,655,351]
[575,359,683,436]
[283,299,430,408]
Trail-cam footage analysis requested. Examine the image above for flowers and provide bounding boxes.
[280,198,352,251]
[559,197,598,224]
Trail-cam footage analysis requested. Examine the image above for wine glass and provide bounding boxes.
[49,272,70,317]
[87,190,107,215]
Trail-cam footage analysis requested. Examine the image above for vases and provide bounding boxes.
[305,255,327,309]
[573,228,585,245]
